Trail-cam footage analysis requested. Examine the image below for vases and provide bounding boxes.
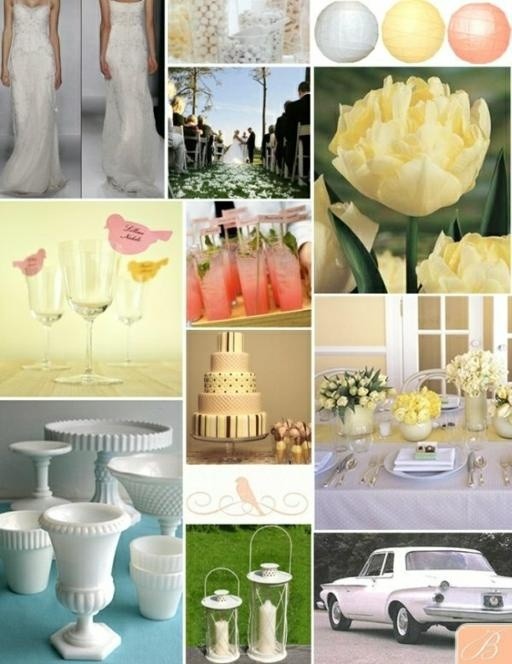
[463,391,486,432]
[338,406,431,440]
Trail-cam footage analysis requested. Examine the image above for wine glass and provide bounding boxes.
[106,453,183,536]
[21,255,69,372]
[51,243,123,386]
[36,502,133,661]
[112,275,149,369]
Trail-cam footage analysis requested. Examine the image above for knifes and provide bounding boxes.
[322,453,353,487]
[466,452,476,487]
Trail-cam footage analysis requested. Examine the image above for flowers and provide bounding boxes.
[445,347,505,395]
[318,368,442,424]
[312,74,510,293]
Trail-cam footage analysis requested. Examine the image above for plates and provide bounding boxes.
[314,445,340,475]
[383,441,469,480]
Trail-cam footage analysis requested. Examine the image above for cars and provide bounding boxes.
[316,548,512,644]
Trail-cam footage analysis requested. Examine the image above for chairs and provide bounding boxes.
[403,368,461,394]
[179,130,224,172]
[260,122,309,184]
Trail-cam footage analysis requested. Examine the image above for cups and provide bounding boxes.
[0,510,53,594]
[128,535,184,621]
[186,246,304,323]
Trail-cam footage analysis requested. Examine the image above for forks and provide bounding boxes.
[500,456,510,487]
[360,456,386,488]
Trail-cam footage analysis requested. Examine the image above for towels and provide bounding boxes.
[392,448,455,472]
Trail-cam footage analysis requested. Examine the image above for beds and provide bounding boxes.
[312,398,510,529]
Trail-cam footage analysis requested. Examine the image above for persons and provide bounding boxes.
[98,0,165,194]
[0,0,68,197]
[168,80,310,194]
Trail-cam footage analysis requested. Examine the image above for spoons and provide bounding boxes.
[335,459,359,486]
[475,456,488,486]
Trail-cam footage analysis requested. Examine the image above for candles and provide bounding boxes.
[214,618,228,655]
[257,600,278,654]
